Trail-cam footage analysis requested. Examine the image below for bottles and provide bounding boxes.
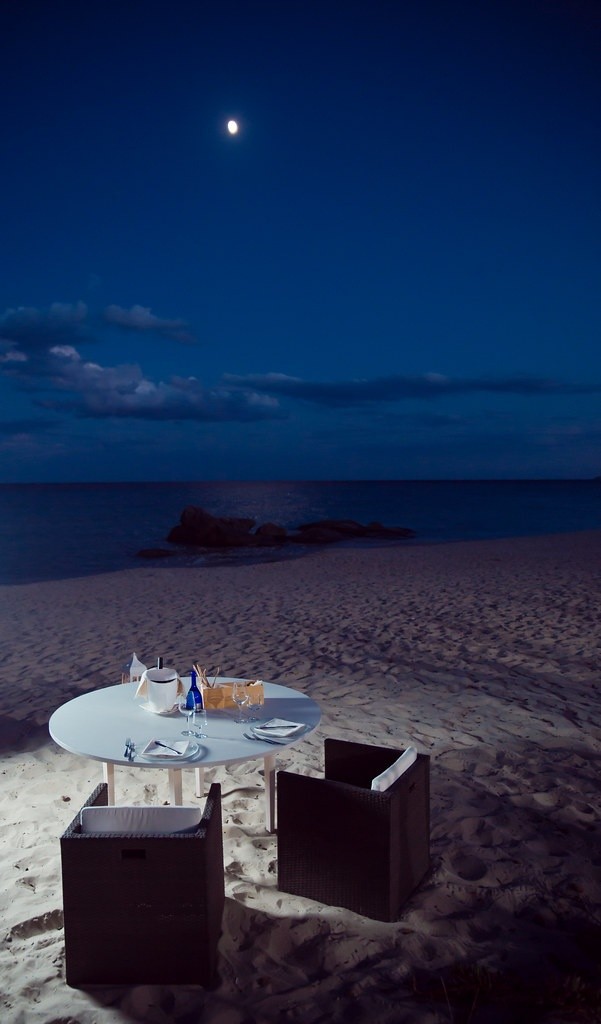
[186,671,203,718]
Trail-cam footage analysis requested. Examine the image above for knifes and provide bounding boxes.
[155,741,182,754]
[254,725,298,729]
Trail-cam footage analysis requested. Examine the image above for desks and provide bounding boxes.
[49,677,320,833]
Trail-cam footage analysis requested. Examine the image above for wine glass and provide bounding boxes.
[247,695,261,720]
[178,692,195,736]
[232,680,249,723]
[192,710,208,739]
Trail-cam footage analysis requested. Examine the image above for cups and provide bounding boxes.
[145,668,180,711]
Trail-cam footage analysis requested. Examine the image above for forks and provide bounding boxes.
[124,738,131,757]
[252,734,275,745]
[128,743,134,761]
[243,732,286,745]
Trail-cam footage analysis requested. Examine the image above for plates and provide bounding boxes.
[254,718,305,737]
[141,739,189,756]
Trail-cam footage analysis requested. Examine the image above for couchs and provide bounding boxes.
[277,738,432,925]
[59,783,224,987]
